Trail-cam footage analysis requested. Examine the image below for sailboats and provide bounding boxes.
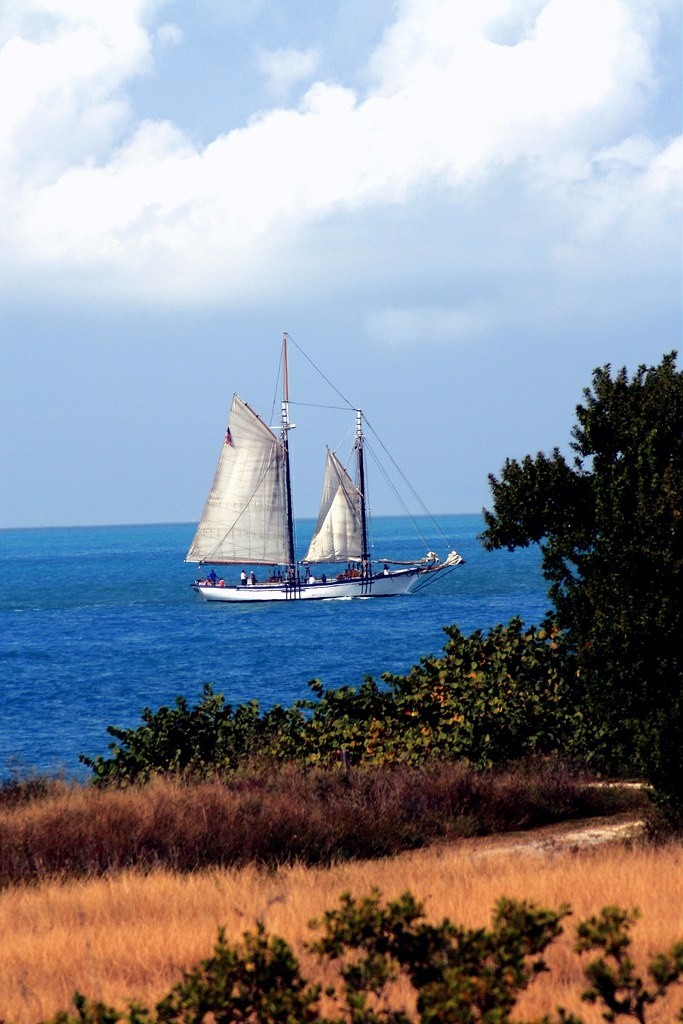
[185,331,469,602]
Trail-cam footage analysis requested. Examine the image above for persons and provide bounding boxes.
[219,577,224,587]
[241,570,255,585]
[308,575,315,584]
[321,574,326,583]
[205,569,218,587]
